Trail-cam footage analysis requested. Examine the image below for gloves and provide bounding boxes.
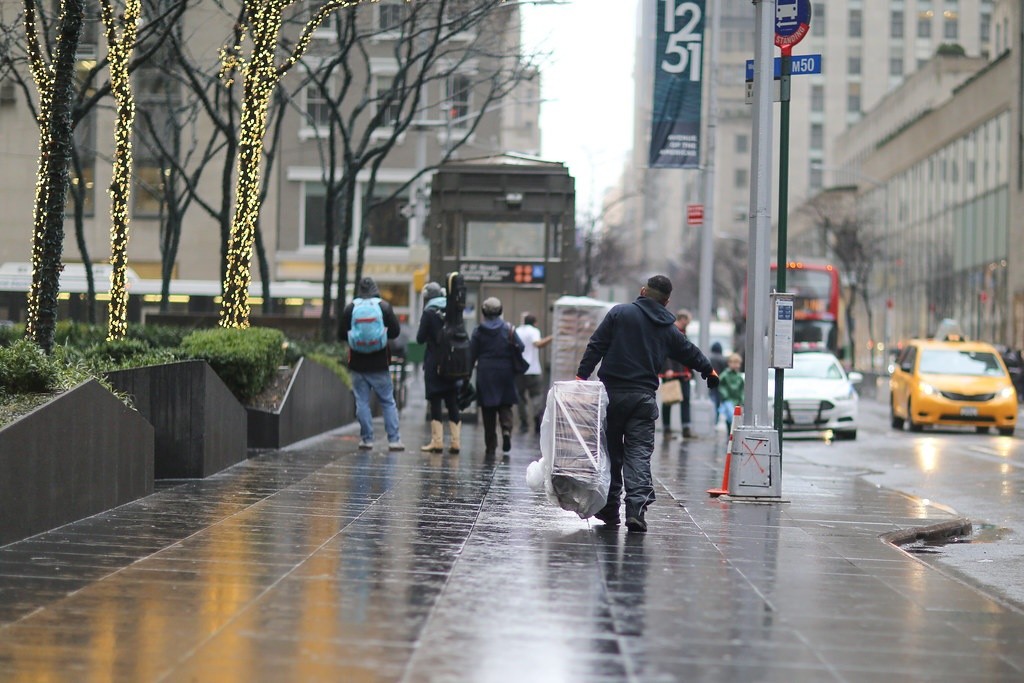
[701,370,719,388]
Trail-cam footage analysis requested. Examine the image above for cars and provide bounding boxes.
[889,334,1017,437]
[767,351,863,440]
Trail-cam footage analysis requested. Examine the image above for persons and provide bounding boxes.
[660,309,690,436]
[471,296,524,450]
[708,342,728,404]
[719,355,744,427]
[417,282,462,452]
[516,315,552,431]
[576,275,718,531]
[336,278,405,449]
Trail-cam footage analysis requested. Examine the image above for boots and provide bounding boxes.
[449,420,462,450]
[421,419,444,452]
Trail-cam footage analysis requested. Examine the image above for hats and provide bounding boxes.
[423,282,444,298]
[481,296,502,316]
[357,277,379,296]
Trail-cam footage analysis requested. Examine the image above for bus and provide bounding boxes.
[743,262,844,368]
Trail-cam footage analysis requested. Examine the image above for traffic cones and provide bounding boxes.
[706,406,743,495]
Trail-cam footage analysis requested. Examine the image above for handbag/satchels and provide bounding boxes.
[508,322,529,373]
[660,379,683,403]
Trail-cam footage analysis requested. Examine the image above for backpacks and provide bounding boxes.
[348,297,387,352]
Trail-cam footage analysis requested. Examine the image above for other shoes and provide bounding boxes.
[595,504,621,524]
[503,430,511,451]
[626,510,647,532]
[664,429,672,440]
[485,448,496,455]
[519,421,528,432]
[359,438,373,448]
[533,414,542,436]
[388,441,404,450]
[683,431,698,439]
